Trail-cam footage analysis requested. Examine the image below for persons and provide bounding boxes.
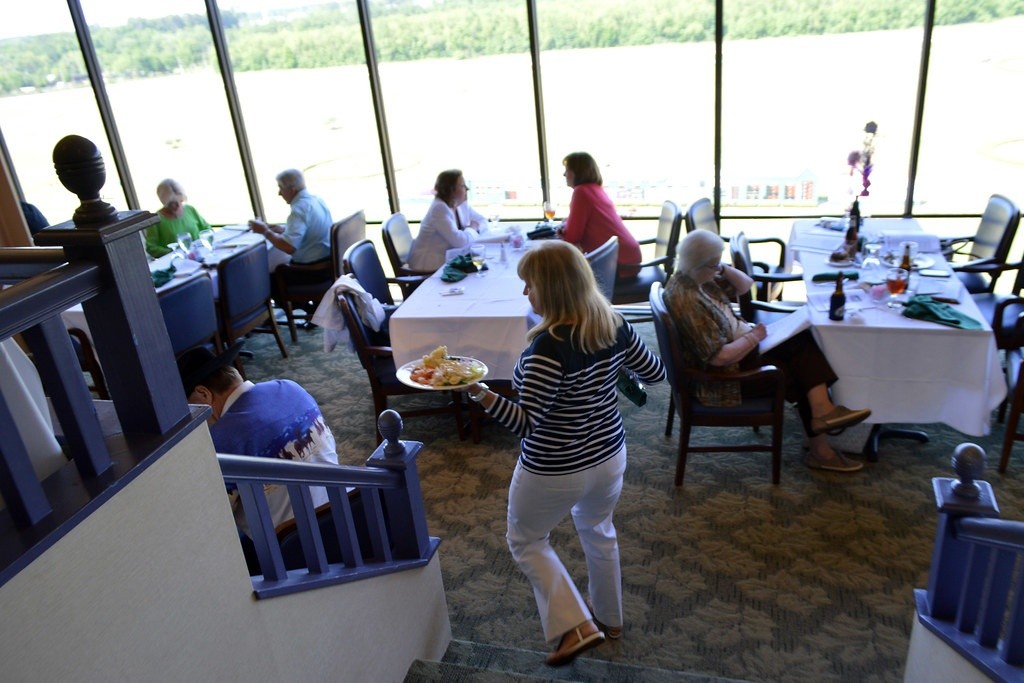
[171,345,348,577]
[552,152,642,286]
[245,169,335,331]
[16,202,50,236]
[662,230,873,473]
[450,240,669,668]
[145,179,214,261]
[407,168,486,292]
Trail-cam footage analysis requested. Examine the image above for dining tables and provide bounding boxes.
[388,222,543,445]
[785,219,994,452]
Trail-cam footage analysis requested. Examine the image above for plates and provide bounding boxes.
[882,250,935,270]
[396,354,488,389]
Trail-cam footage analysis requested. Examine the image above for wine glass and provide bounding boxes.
[200,228,220,258]
[886,267,909,308]
[544,201,559,224]
[862,242,882,284]
[471,244,489,278]
[167,242,184,267]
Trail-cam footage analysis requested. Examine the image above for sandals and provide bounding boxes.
[545,619,605,668]
[589,610,623,639]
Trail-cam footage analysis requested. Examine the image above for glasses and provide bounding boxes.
[703,264,719,270]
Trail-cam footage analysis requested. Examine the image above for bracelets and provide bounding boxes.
[743,333,756,348]
[465,383,490,402]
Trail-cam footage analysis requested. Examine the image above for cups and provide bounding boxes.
[177,229,193,254]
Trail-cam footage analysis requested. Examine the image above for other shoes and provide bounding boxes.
[811,405,871,432]
[804,448,863,472]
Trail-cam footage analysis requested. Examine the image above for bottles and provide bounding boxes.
[845,215,858,260]
[828,270,846,321]
[897,244,911,293]
[850,195,860,233]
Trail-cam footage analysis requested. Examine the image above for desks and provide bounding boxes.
[61,223,290,359]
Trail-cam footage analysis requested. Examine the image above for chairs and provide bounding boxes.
[157,271,225,356]
[332,273,466,450]
[938,194,1021,294]
[685,197,790,302]
[996,346,1024,473]
[274,209,366,342]
[729,231,807,325]
[951,253,1024,422]
[608,200,683,323]
[380,213,437,303]
[646,279,785,490]
[584,235,619,305]
[206,237,289,383]
[342,239,433,318]
